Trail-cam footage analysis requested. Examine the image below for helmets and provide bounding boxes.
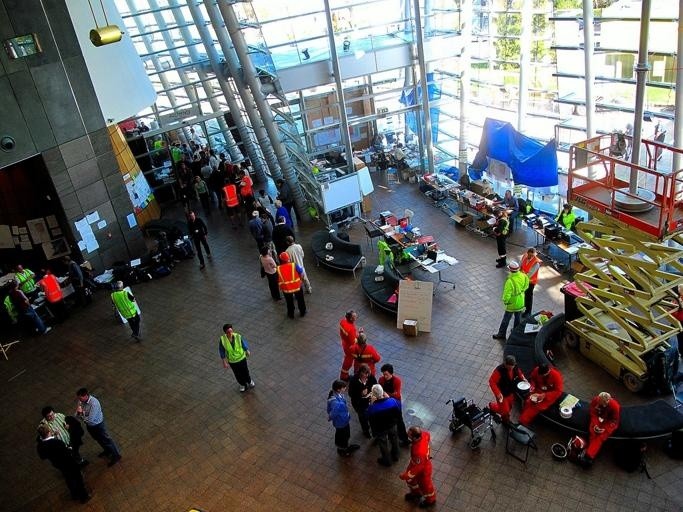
[278,251,292,261]
[551,442,567,461]
[325,242,333,250]
[507,261,521,272]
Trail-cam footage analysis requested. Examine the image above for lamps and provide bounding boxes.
[343,40,350,52]
[302,48,310,60]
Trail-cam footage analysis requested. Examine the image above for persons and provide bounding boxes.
[36,388,120,504]
[489,355,528,420]
[368,384,400,466]
[519,247,543,318]
[555,204,576,231]
[493,262,529,339]
[350,333,381,375]
[348,364,377,438]
[111,281,144,340]
[327,380,359,458]
[219,324,255,392]
[395,143,410,161]
[124,121,311,318]
[370,133,381,150]
[340,310,364,381]
[493,208,510,268]
[581,392,620,467]
[518,363,564,426]
[379,364,409,447]
[493,190,519,234]
[10,257,84,335]
[399,426,437,509]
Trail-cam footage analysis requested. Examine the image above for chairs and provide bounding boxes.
[391,262,412,280]
[668,373,683,411]
[502,420,538,464]
[363,224,385,253]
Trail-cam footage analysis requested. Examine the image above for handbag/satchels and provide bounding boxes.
[259,266,265,278]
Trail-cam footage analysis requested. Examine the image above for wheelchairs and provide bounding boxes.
[444,395,500,449]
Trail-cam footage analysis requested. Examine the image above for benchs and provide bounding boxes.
[361,265,400,320]
[503,309,683,440]
[311,221,362,280]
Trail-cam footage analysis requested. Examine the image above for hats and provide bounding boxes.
[506,354,518,365]
[251,211,258,217]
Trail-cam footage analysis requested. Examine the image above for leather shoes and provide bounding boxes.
[199,265,205,271]
[207,251,211,258]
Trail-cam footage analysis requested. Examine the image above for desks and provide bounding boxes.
[31,298,55,324]
[421,174,461,201]
[457,191,514,219]
[371,221,459,296]
[521,216,594,273]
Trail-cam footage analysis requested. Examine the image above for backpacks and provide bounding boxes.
[566,434,587,464]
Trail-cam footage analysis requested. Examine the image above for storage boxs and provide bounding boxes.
[403,319,418,337]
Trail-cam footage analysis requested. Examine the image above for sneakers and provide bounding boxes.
[248,380,255,387]
[493,333,506,339]
[42,327,54,336]
[131,333,140,342]
[297,309,308,317]
[97,450,108,458]
[239,384,245,391]
[377,456,393,468]
[419,498,436,507]
[348,444,361,450]
[106,455,121,467]
[495,256,506,269]
[286,310,295,319]
[404,491,421,500]
[305,286,313,294]
[337,448,350,458]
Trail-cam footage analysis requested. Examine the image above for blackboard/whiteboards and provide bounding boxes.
[320,172,362,215]
[396,280,433,332]
